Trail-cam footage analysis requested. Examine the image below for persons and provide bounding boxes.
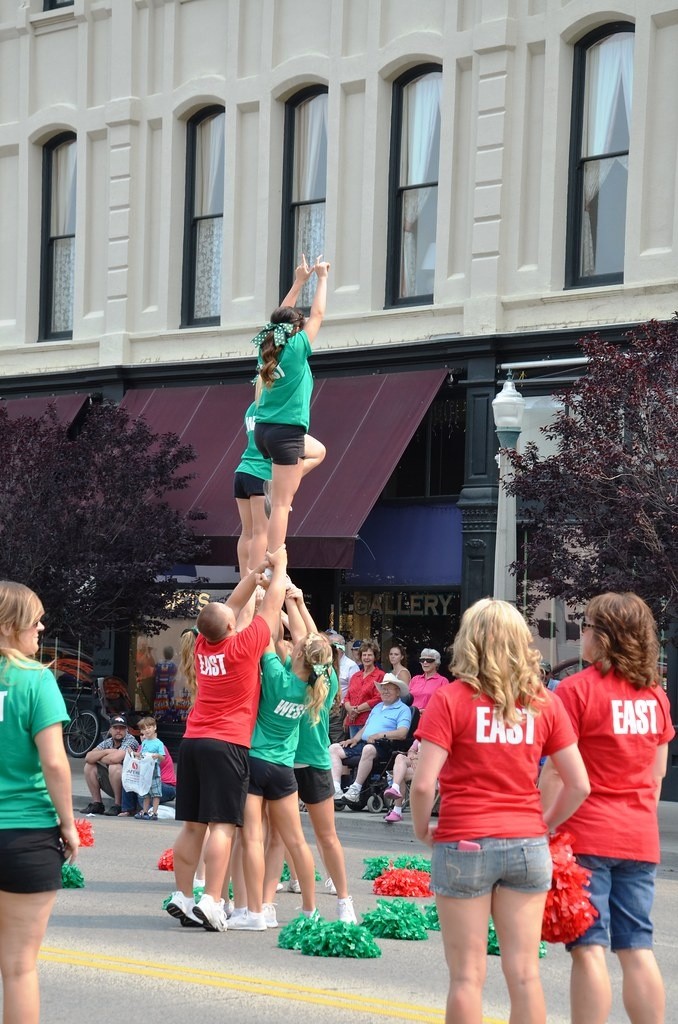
[166,544,358,932]
[409,596,591,1024]
[136,638,177,711]
[118,717,176,820]
[0,579,80,1024]
[79,715,139,816]
[553,591,675,1024]
[233,375,292,598]
[322,632,561,823]
[253,253,330,592]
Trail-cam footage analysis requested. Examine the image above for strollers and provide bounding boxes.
[96,677,153,745]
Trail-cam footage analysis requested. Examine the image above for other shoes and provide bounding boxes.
[135,810,158,820]
[262,569,297,590]
[80,803,104,813]
[118,810,134,817]
[264,479,293,515]
[105,805,119,816]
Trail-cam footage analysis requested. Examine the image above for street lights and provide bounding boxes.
[490,369,525,608]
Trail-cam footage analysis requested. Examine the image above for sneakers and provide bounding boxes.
[226,909,266,931]
[262,902,278,927]
[337,898,357,923]
[325,876,337,895]
[286,880,301,892]
[341,786,360,804]
[193,894,228,931]
[332,786,343,800]
[168,890,202,927]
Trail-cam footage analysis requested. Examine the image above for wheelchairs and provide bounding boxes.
[334,693,420,813]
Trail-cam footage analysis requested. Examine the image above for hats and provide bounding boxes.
[111,716,127,727]
[351,641,364,650]
[374,673,410,697]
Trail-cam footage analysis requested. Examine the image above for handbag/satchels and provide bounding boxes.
[122,750,158,796]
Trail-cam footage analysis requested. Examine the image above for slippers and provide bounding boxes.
[383,788,402,800]
[385,812,402,822]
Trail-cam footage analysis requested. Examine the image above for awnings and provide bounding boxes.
[0,369,446,569]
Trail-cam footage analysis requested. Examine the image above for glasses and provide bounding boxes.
[419,658,438,664]
[581,616,601,634]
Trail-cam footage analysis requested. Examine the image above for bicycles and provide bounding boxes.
[63,679,100,758]
